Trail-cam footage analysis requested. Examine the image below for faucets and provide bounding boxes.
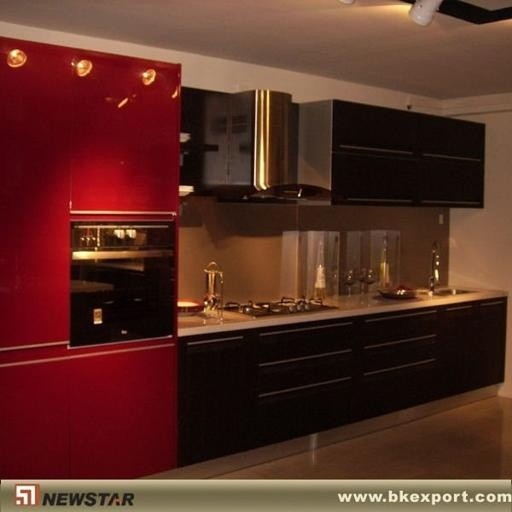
[432,269,443,291]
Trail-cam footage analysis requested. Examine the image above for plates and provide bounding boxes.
[377,288,420,297]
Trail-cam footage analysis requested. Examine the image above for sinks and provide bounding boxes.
[436,288,469,296]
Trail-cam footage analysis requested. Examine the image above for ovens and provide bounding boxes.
[68,220,175,349]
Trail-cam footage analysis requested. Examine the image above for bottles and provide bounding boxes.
[201,269,223,321]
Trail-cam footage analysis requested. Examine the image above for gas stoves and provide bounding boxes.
[219,299,340,318]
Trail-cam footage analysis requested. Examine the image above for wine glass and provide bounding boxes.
[341,268,379,300]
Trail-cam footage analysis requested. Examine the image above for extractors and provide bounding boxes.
[210,85,334,206]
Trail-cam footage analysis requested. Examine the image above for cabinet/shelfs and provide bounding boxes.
[438,289,512,403]
[297,99,488,211]
[249,298,443,450]
[177,322,250,469]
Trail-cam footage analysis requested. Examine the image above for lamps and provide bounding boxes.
[135,66,158,87]
[64,53,94,79]
[403,0,445,26]
[0,45,28,70]
